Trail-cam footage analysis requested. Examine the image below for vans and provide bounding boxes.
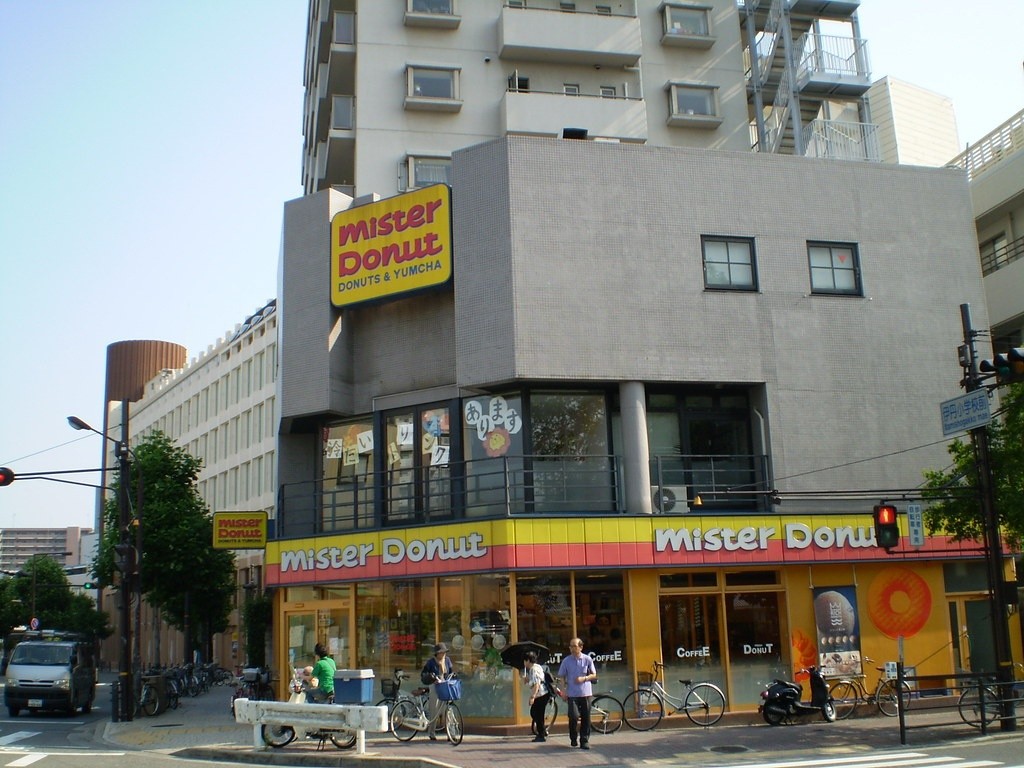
[3,640,96,717]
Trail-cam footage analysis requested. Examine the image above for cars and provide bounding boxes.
[459,610,511,645]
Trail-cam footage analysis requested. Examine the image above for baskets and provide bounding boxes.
[435,679,461,700]
[637,671,654,687]
[243,672,262,682]
[381,679,397,699]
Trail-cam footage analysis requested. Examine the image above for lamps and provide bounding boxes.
[694,492,703,505]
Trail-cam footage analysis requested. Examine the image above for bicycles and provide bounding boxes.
[956,661,1024,728]
[829,655,911,720]
[623,660,725,731]
[117,663,233,719]
[531,678,625,735]
[230,665,275,718]
[375,668,464,746]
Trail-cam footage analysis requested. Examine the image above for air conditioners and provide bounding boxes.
[651,485,688,514]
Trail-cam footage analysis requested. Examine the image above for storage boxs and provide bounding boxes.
[333,668,376,704]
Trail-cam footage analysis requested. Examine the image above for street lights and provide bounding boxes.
[68,415,133,722]
[31,551,72,631]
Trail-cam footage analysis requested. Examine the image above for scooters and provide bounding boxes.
[757,664,836,726]
[261,679,356,751]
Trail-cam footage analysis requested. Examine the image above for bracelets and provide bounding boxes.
[531,696,534,699]
[584,676,588,682]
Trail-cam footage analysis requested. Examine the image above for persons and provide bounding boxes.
[420,642,460,742]
[14,648,40,663]
[558,638,597,750]
[56,649,68,663]
[297,642,337,737]
[523,651,551,742]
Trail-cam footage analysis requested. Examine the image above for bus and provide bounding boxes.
[4,630,100,669]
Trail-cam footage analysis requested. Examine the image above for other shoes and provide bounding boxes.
[532,730,548,742]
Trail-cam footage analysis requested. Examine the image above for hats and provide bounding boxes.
[434,643,449,655]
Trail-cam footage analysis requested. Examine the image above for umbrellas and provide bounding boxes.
[501,641,551,684]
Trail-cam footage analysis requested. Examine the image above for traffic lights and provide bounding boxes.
[84,583,98,589]
[1004,580,1019,604]
[126,573,146,593]
[872,504,900,548]
[0,467,14,486]
[980,347,1024,383]
[111,543,133,575]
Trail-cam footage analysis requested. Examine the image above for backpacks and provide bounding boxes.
[540,668,558,697]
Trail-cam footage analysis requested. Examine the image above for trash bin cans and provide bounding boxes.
[140,676,167,715]
[235,665,243,677]
